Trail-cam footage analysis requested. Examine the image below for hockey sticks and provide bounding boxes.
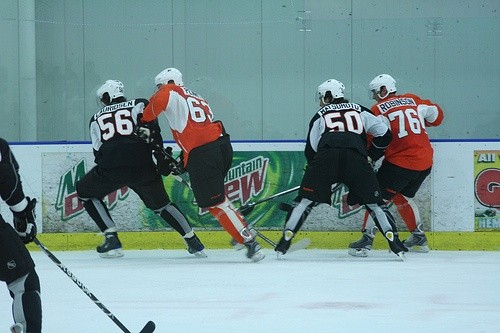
[279,158,371,213]
[168,170,311,253]
[238,185,301,215]
[32,236,157,333]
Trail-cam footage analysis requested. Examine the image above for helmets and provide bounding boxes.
[154,68,184,87]
[97,80,125,102]
[369,73,397,99]
[317,79,345,104]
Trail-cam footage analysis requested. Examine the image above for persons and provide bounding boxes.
[142,68,265,262]
[347,74,443,258]
[273,79,408,262]
[77,79,207,257]
[0,138,43,333]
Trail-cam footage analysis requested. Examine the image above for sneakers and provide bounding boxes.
[386,231,409,262]
[402,223,428,253]
[231,219,257,251]
[274,229,295,260]
[348,226,378,257]
[245,241,265,262]
[97,231,124,259]
[184,232,207,258]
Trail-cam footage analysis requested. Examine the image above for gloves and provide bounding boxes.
[9,196,37,244]
[152,146,173,176]
[169,151,186,176]
[134,113,158,139]
